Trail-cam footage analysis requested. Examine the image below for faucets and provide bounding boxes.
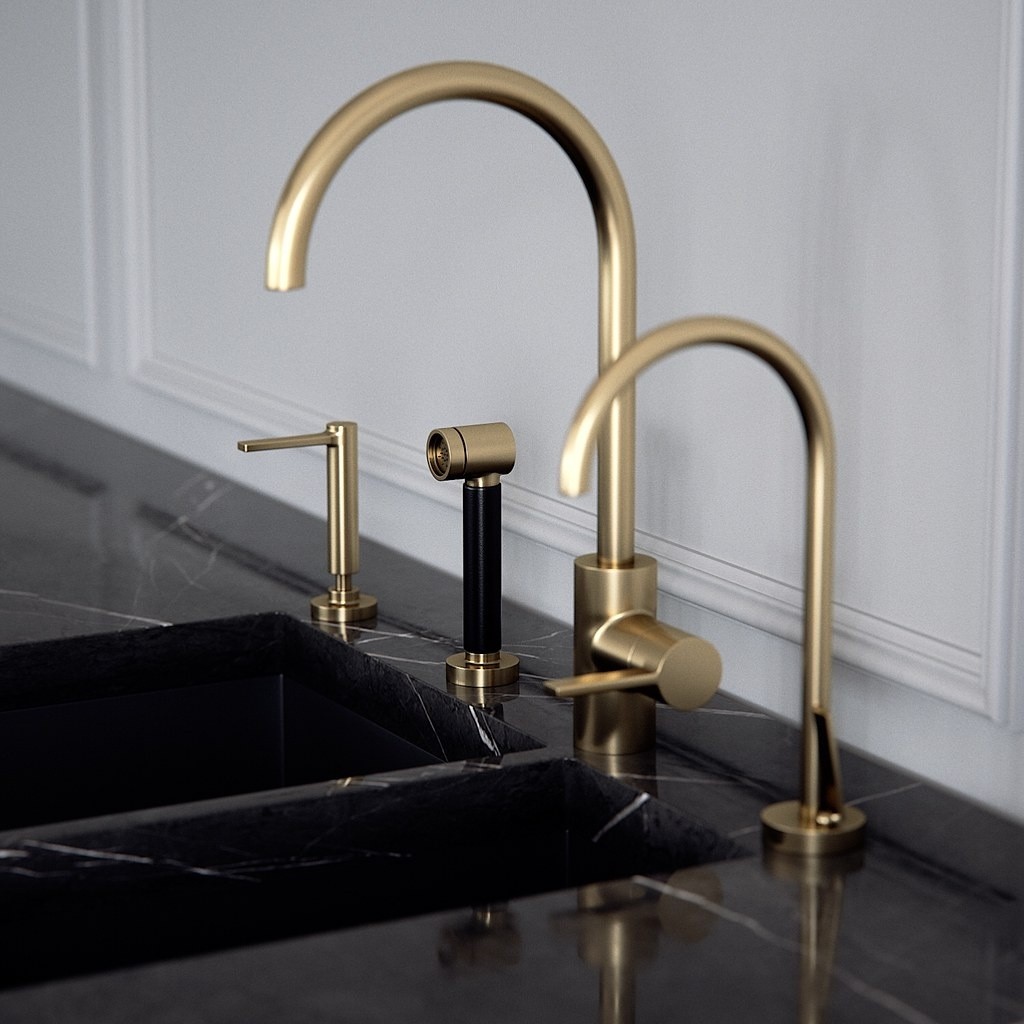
[556,313,871,859]
[264,57,722,754]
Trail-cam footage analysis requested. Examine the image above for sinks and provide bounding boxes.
[1,609,541,831]
[3,759,727,1000]
